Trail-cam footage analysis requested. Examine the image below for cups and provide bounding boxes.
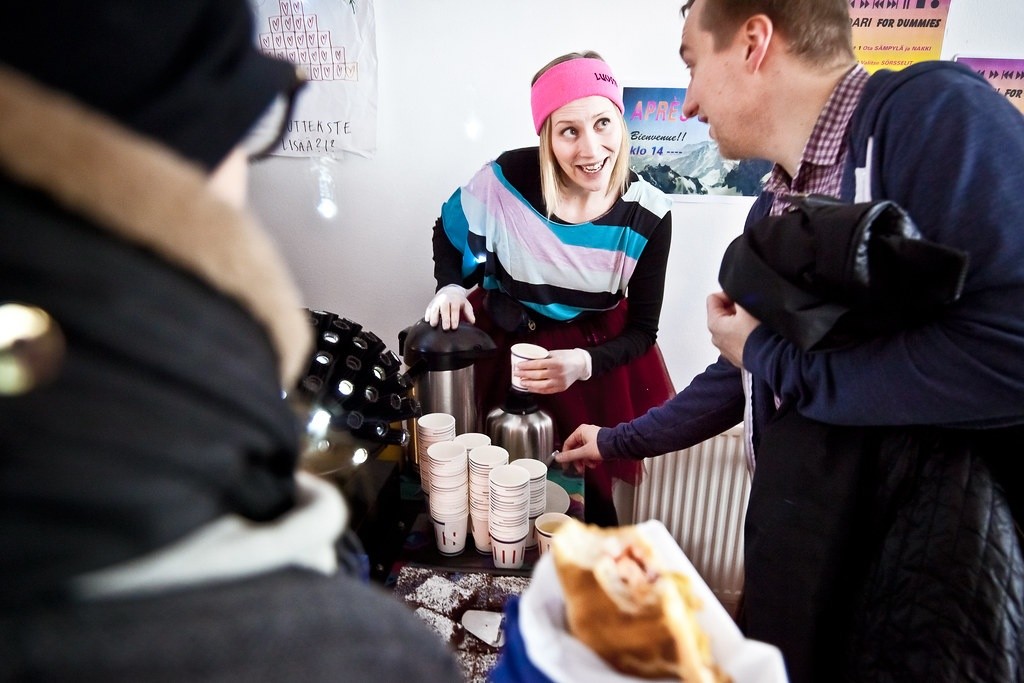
[535,513,572,557]
[510,343,550,392]
[418,413,548,569]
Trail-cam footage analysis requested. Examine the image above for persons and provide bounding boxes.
[424,50,677,527]
[0,1,471,683]
[559,0,1024,683]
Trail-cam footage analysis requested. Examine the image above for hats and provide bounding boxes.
[0,0,280,177]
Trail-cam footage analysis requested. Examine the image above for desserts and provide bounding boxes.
[396,566,534,683]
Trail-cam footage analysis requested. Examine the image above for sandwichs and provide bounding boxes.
[550,518,738,683]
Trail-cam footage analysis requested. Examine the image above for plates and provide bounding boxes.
[547,479,570,514]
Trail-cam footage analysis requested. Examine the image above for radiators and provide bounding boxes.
[628,422,754,606]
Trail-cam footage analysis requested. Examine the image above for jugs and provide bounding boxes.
[399,318,497,474]
[485,398,560,468]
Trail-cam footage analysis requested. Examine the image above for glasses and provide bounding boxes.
[242,65,307,165]
